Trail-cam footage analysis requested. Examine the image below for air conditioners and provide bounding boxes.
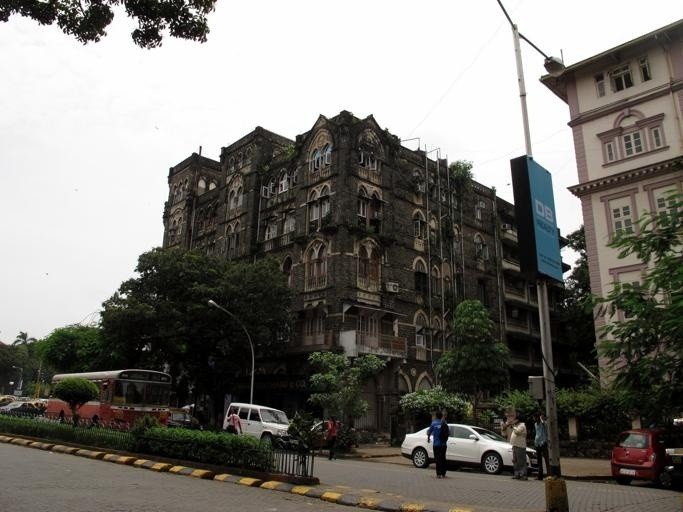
[386,282,399,293]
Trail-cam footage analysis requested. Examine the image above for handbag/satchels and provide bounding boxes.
[323,431,333,441]
[226,424,236,434]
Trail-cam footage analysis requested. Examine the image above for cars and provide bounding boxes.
[300,421,361,447]
[608,426,683,488]
[169,411,204,431]
[0,393,48,418]
[399,423,538,475]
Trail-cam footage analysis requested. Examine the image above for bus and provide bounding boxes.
[46,368,172,429]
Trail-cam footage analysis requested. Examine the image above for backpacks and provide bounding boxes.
[438,419,450,442]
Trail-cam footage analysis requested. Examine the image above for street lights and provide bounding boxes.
[11,365,24,397]
[207,299,256,407]
[504,23,582,512]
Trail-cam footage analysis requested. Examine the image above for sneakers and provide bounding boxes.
[534,477,543,480]
[519,477,528,481]
[510,475,521,480]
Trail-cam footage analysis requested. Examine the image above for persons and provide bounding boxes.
[509,414,527,481]
[500,417,509,439]
[325,415,338,460]
[533,411,549,480]
[427,411,447,479]
[226,409,242,434]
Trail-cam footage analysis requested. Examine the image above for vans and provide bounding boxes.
[221,401,293,451]
